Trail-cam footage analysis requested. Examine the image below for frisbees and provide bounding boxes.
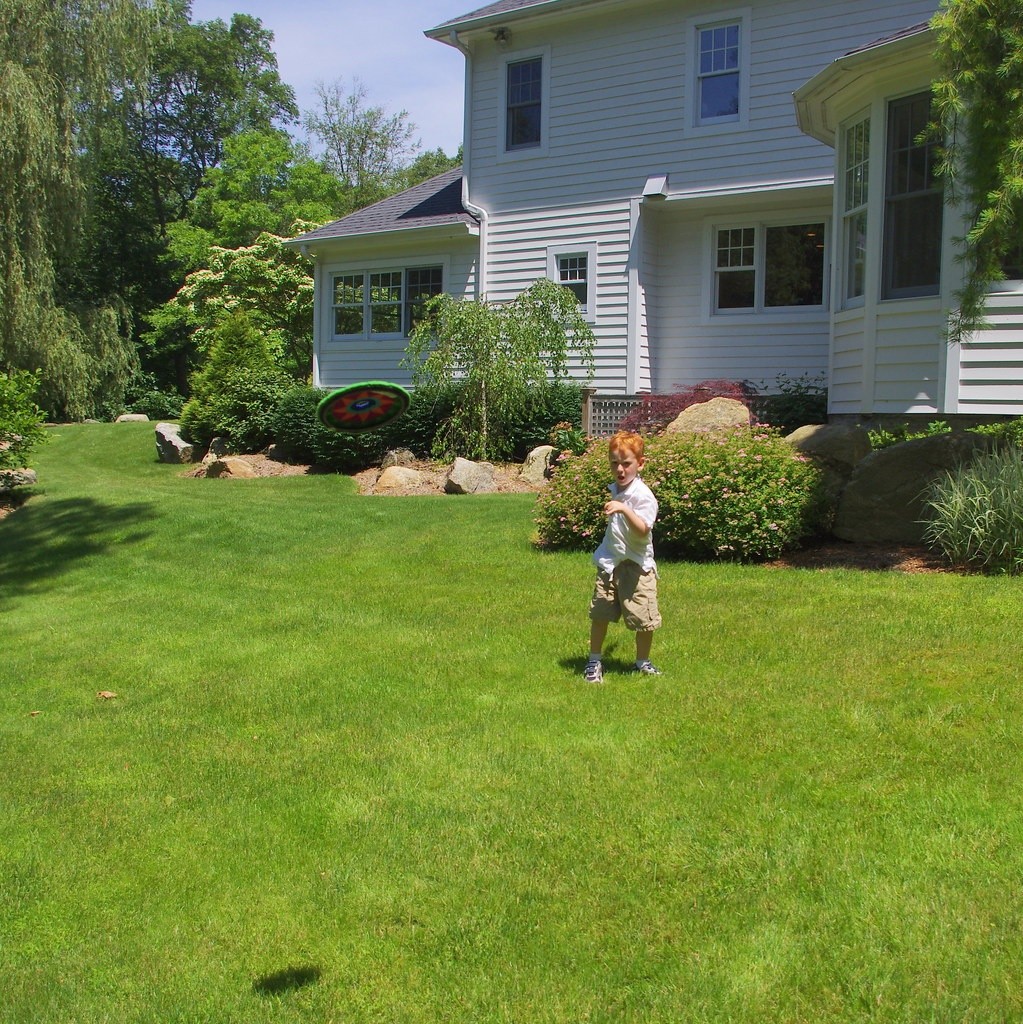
[313,378,413,434]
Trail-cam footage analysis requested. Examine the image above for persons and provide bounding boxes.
[584,431,662,683]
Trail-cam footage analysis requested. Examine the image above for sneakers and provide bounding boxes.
[633,661,662,674]
[584,660,603,684]
